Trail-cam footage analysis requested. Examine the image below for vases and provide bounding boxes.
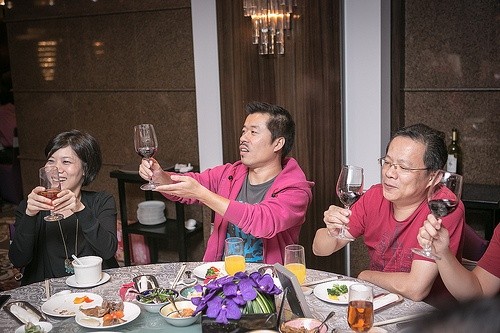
[202,290,277,333]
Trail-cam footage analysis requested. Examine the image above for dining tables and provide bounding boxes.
[0,261,436,333]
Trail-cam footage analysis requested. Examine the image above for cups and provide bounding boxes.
[225,237,246,276]
[284,245,374,333]
[72,256,103,286]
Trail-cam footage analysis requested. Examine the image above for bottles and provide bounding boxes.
[446,127,462,176]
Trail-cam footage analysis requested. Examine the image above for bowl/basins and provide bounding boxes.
[135,288,180,313]
[178,284,209,301]
[159,301,202,327]
[279,318,328,333]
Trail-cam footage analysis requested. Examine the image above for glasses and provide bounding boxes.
[379,157,429,174]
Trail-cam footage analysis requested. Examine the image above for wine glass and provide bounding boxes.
[39,166,64,221]
[411,169,463,261]
[134,123,160,190]
[331,164,364,241]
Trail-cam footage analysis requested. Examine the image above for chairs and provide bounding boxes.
[110,166,197,265]
[464,224,487,260]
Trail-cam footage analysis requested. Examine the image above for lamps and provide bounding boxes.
[242,0,299,55]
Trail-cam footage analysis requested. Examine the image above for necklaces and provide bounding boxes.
[58,219,78,274]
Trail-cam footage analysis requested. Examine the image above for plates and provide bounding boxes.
[15,322,53,333]
[313,280,371,304]
[192,262,228,280]
[137,200,166,225]
[66,271,111,288]
[41,292,103,317]
[75,302,141,329]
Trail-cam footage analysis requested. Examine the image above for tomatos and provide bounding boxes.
[206,268,215,275]
[103,311,124,321]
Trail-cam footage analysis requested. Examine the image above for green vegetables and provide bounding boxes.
[327,284,349,296]
[140,287,173,304]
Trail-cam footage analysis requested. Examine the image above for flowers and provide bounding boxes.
[200,275,276,320]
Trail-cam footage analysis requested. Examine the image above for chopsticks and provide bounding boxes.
[373,312,423,327]
[45,280,50,300]
[170,265,186,289]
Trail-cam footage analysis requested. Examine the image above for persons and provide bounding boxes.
[139,101,313,264]
[0,99,18,163]
[311,123,500,333]
[8,131,121,285]
